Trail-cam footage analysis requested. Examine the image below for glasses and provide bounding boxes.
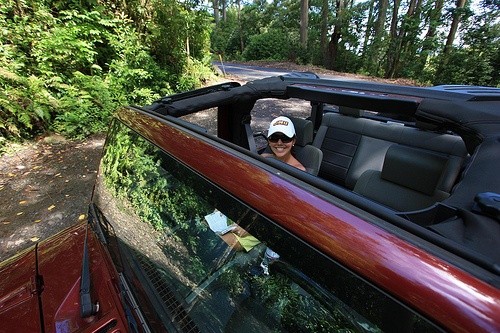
[269,133,295,143]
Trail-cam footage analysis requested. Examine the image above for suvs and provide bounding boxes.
[0,72,499,333]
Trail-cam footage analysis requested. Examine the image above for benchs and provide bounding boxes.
[312,107,467,193]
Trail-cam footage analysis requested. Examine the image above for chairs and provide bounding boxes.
[265,115,322,181]
[351,144,465,228]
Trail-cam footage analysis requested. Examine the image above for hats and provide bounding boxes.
[268,116,296,138]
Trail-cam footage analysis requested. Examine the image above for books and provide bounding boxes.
[204,210,237,235]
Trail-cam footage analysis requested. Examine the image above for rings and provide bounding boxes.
[237,231,240,234]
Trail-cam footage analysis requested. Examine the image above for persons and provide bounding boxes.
[205,114,307,251]
